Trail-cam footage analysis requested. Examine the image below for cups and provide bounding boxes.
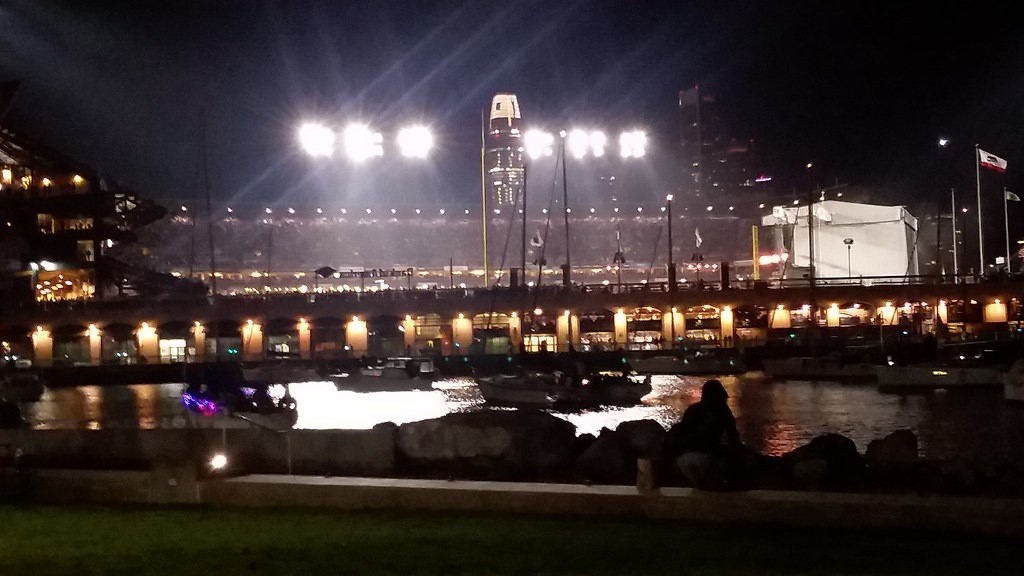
[637,458,659,496]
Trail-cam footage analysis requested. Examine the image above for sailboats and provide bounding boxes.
[754,162,897,381]
[179,104,298,431]
[636,193,745,376]
[472,129,654,411]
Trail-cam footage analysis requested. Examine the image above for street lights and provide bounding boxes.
[666,193,676,297]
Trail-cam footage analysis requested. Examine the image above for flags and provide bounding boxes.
[1006,191,1021,201]
[979,149,1007,174]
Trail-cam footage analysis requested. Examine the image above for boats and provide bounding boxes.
[328,368,350,378]
[360,365,383,377]
[877,333,1024,402]
[0,354,45,404]
